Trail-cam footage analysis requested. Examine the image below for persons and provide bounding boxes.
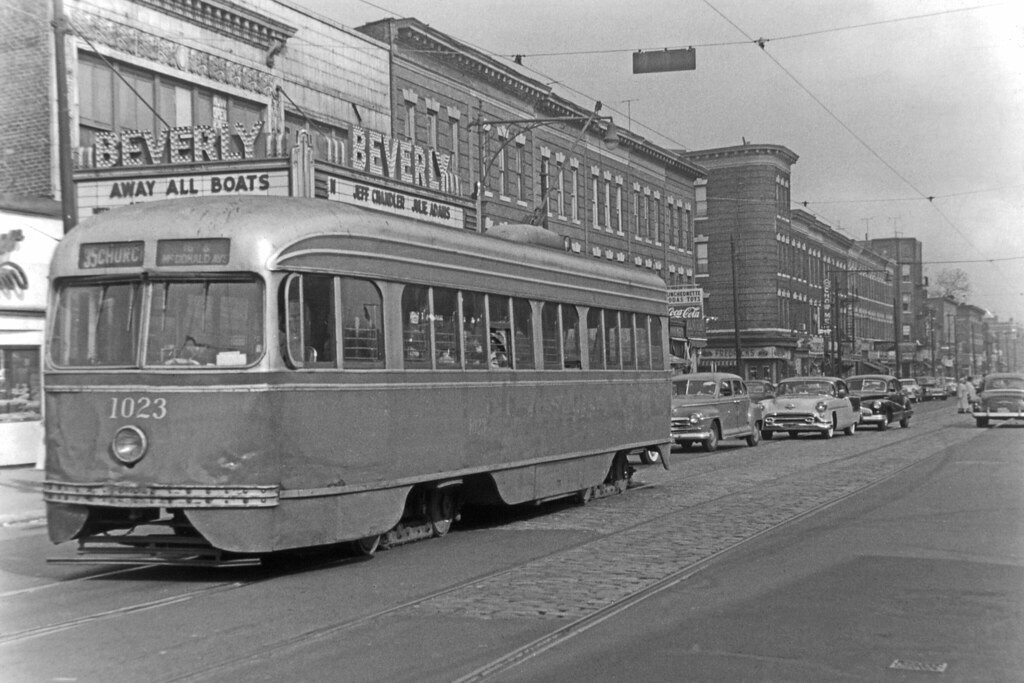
[491,332,508,367]
[958,376,976,413]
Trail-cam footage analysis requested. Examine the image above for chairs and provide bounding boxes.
[344,321,375,361]
[515,332,529,363]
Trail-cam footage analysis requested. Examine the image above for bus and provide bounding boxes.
[41,196,672,568]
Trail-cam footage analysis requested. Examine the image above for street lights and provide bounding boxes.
[825,270,892,376]
[477,112,619,233]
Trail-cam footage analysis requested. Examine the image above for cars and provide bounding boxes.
[671,372,763,452]
[917,376,947,400]
[844,375,915,429]
[898,379,922,402]
[755,376,860,438]
[970,373,1024,426]
[743,380,775,402]
[936,376,957,394]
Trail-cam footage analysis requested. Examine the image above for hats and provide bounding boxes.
[490,332,506,354]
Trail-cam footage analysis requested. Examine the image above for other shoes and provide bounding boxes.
[958,410,971,413]
